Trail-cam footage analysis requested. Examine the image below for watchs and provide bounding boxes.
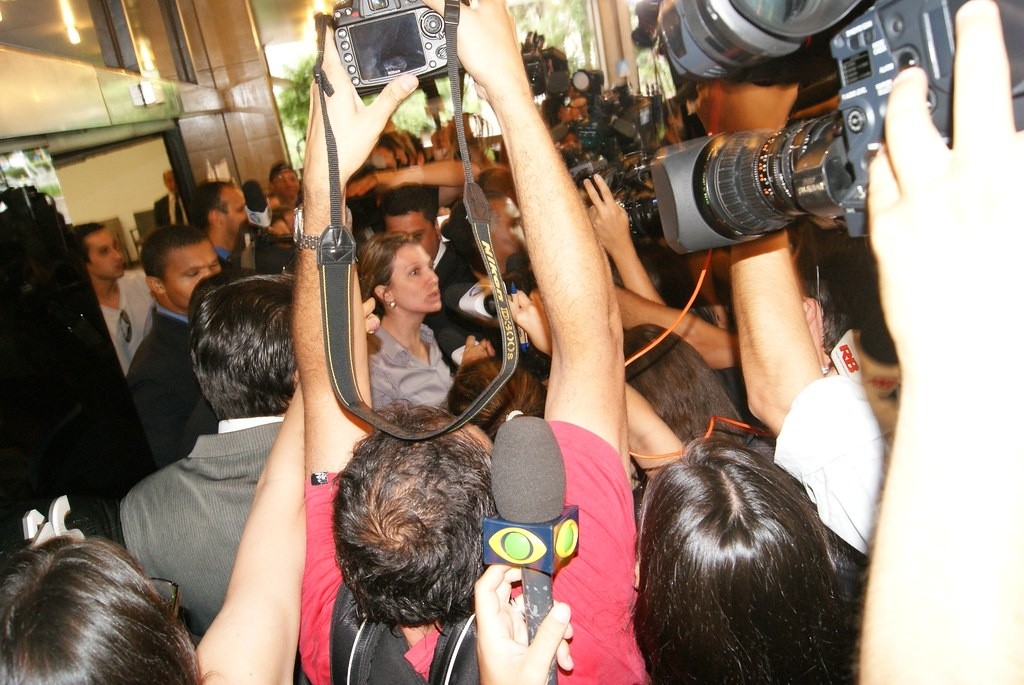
[293,204,352,250]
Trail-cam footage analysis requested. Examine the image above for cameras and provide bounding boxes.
[331,0,467,95]
[521,31,666,240]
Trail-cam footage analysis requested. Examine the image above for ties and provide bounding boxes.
[175,193,185,226]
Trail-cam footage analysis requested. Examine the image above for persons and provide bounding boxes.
[632,436,862,685]
[474,564,574,685]
[70,81,903,556]
[295,0,638,685]
[120,275,300,637]
[789,0,1024,685]
[0,382,306,685]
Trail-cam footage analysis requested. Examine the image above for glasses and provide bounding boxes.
[148,575,181,619]
[119,308,132,344]
[275,175,298,182]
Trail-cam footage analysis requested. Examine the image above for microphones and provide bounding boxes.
[443,283,500,320]
[241,179,273,236]
[828,304,900,399]
[479,415,579,685]
